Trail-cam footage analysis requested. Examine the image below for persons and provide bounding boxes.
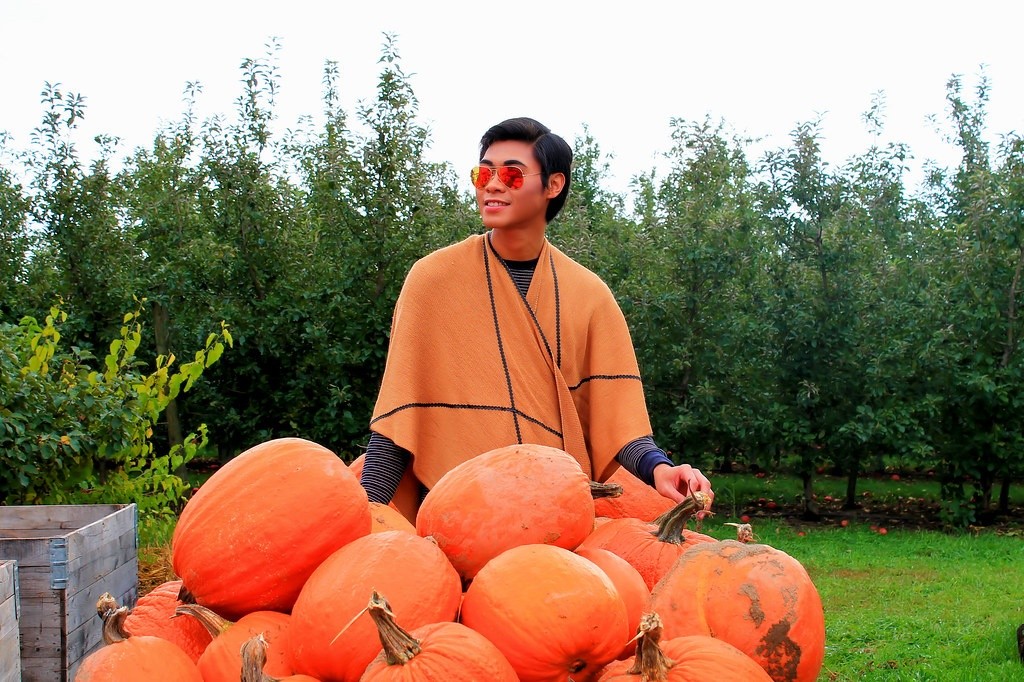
[360,118,713,521]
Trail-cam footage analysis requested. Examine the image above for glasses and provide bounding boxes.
[470,166,543,190]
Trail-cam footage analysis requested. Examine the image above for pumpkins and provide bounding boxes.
[73,437,826,682]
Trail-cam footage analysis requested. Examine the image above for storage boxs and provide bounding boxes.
[0,503,137,682]
